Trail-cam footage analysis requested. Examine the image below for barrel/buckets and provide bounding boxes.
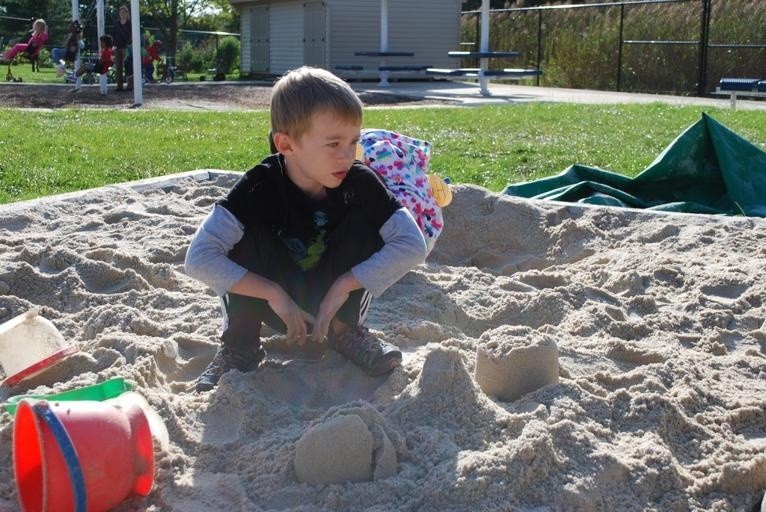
[0,310,80,385]
[12,391,153,512]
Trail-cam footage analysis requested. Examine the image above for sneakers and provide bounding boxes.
[64,72,76,81]
[324,322,404,375]
[195,337,270,394]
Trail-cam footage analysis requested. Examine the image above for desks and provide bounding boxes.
[447,50,521,96]
[354,50,414,88]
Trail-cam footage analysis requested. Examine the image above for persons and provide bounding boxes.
[52,5,161,83]
[183,65,430,394]
[268,128,452,259]
[0,19,48,62]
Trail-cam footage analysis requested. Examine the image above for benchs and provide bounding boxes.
[483,67,542,81]
[377,65,433,72]
[333,64,364,72]
[425,67,482,77]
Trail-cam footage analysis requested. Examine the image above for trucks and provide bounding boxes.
[718,76,766,100]
